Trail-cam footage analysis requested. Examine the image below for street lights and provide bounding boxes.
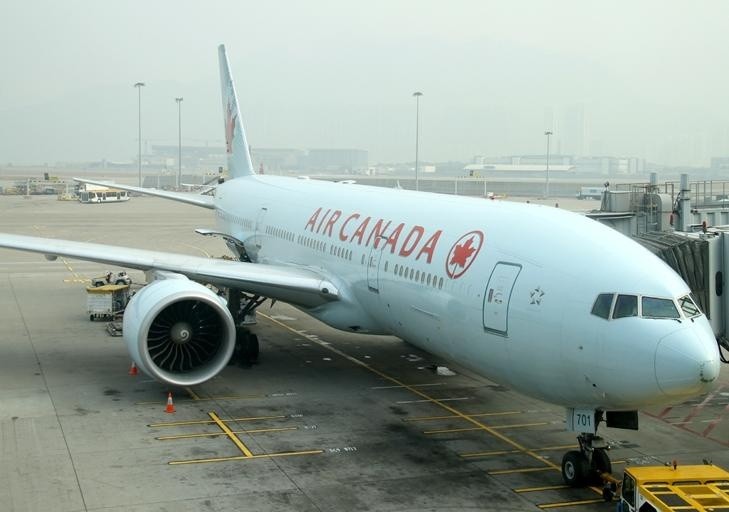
[544,131,552,201]
[413,92,424,191]
[134,81,145,188]
[175,96,183,191]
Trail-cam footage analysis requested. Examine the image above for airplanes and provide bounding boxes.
[0,44,721,488]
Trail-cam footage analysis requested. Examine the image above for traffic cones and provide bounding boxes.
[163,392,176,413]
[128,360,138,376]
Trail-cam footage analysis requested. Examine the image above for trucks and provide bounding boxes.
[575,186,610,200]
[92,270,132,287]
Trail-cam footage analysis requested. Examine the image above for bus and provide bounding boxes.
[78,189,131,204]
